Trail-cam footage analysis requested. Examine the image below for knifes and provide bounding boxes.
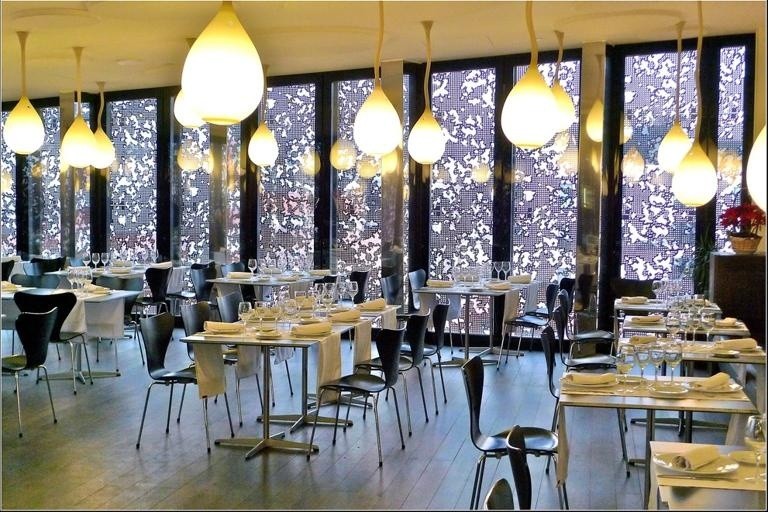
[658,474,740,481]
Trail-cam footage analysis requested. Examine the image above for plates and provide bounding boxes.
[469,287,488,292]
[710,345,762,358]
[559,378,743,396]
[715,321,743,327]
[626,318,662,324]
[654,453,739,474]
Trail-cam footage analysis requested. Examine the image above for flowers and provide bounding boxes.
[719,204,766,232]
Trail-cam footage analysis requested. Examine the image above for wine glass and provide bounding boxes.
[615,345,682,393]
[744,414,767,485]
[238,281,359,339]
[247,258,276,280]
[453,261,510,289]
[67,268,93,297]
[653,278,716,348]
[82,252,116,272]
[141,249,159,268]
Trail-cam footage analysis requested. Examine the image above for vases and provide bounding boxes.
[728,234,762,254]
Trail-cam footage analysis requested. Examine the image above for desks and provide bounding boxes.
[709,253,767,352]
